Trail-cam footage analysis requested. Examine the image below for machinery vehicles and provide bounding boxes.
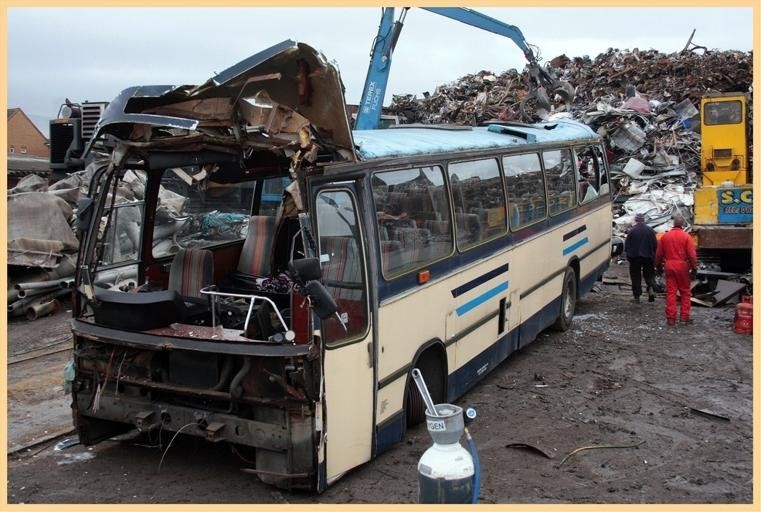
[693,90,754,269]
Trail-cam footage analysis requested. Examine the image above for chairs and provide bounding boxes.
[409,210,441,228]
[380,241,407,277]
[379,226,389,242]
[393,227,432,267]
[317,236,362,301]
[455,207,462,213]
[509,203,519,227]
[532,195,545,218]
[470,207,501,237]
[456,213,482,245]
[548,190,561,214]
[509,198,534,224]
[559,191,575,211]
[236,216,276,278]
[383,219,417,237]
[168,248,214,308]
[424,220,451,258]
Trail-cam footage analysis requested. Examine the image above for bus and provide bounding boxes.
[71,40,612,494]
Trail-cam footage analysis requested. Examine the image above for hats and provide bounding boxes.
[635,213,645,222]
[674,213,684,226]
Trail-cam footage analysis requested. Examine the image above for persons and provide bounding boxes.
[654,216,698,326]
[625,213,658,302]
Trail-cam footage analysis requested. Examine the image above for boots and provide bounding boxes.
[635,288,640,299]
[649,286,654,302]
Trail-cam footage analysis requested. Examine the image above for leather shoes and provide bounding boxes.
[668,318,675,324]
[680,319,693,325]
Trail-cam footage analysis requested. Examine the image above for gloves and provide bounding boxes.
[692,271,696,280]
[656,267,662,277]
